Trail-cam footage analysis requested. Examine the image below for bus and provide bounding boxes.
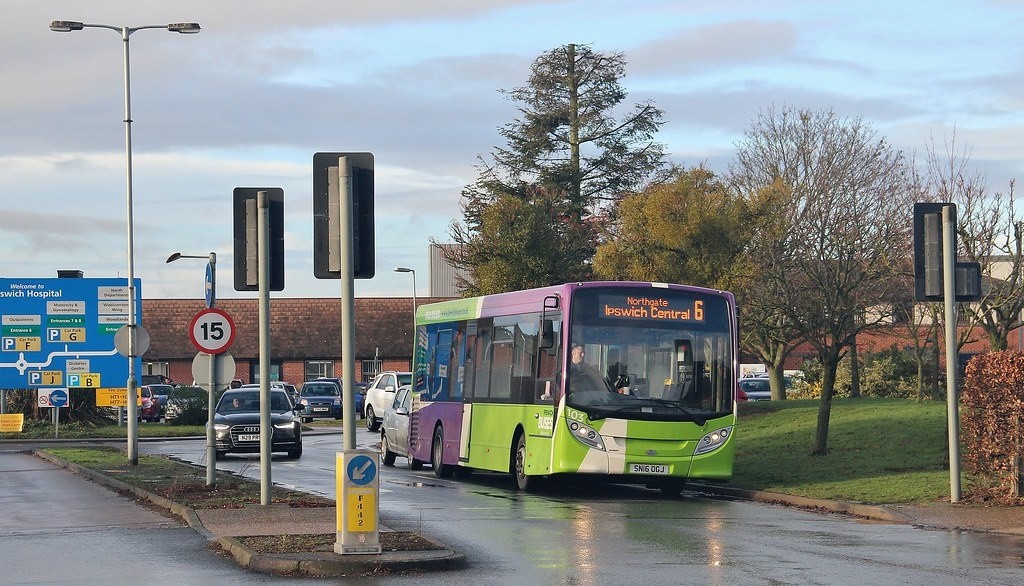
[409,281,741,498]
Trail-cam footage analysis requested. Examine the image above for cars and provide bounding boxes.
[378,385,431,470]
[141,375,245,424]
[205,377,366,459]
[738,370,805,401]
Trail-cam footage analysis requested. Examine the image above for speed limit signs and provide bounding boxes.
[188,308,236,355]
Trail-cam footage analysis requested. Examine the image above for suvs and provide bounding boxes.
[364,371,412,432]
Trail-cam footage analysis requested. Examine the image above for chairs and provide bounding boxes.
[324,387,329,393]
[307,387,313,393]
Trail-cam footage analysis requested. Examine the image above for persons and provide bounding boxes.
[567,343,623,396]
[164,378,170,385]
[229,397,245,410]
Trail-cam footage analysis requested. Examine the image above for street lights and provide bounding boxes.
[166,252,217,485]
[394,267,417,332]
[47,19,203,464]
